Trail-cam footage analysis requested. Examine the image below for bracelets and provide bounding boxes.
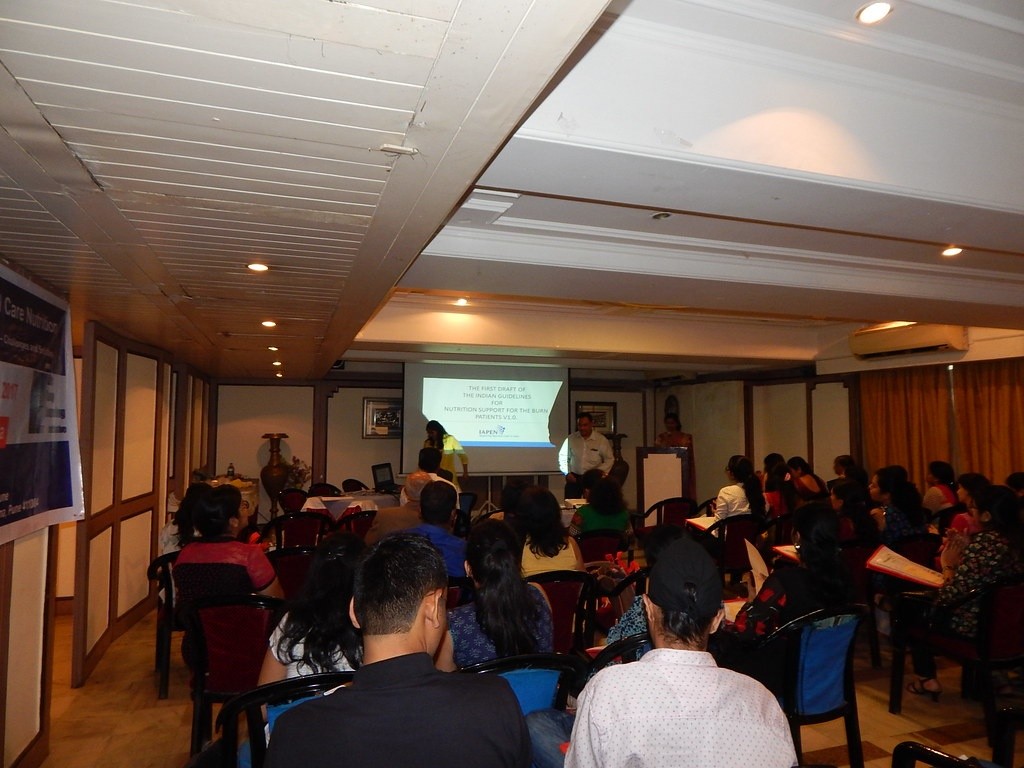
[941,566,953,573]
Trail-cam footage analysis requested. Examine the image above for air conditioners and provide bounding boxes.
[848,320,970,363]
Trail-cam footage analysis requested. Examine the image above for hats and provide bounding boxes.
[649,538,722,619]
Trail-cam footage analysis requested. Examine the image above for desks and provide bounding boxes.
[301,488,399,520]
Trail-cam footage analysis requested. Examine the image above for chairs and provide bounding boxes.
[887,572,1023,716]
[749,601,869,768]
[891,704,1024,768]
[146,479,796,766]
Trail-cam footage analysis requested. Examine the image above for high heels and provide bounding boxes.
[907,678,943,702]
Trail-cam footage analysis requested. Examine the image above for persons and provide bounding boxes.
[174,480,286,673]
[654,412,697,505]
[557,412,615,499]
[710,452,1024,702]
[157,448,727,768]
[264,534,535,768]
[734,502,857,655]
[424,420,469,509]
[524,541,800,768]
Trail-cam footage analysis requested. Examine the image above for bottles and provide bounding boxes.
[227,462,234,479]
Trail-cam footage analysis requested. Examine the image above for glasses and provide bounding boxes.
[725,466,730,471]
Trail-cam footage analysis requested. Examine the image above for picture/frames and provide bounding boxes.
[362,397,403,440]
[576,401,619,433]
